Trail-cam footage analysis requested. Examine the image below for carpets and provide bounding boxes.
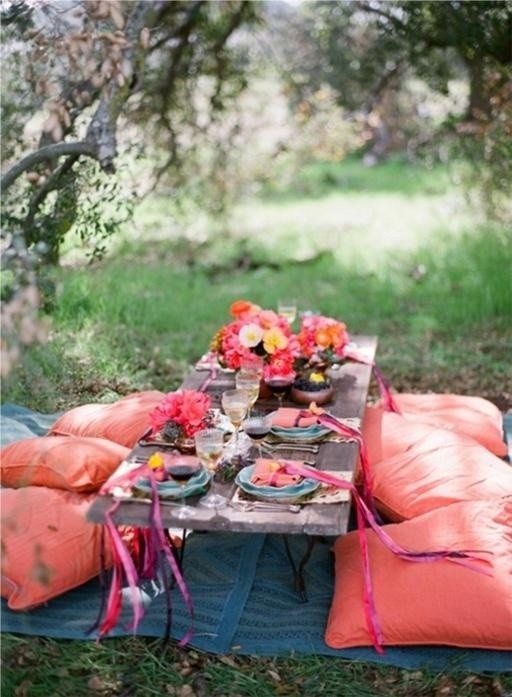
[0,399,512,672]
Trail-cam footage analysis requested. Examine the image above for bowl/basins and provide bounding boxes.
[291,383,333,406]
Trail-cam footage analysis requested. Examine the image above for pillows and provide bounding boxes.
[361,404,512,520]
[323,496,509,652]
[377,395,510,457]
[1,389,167,614]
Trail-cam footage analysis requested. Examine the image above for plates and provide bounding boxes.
[234,463,323,505]
[261,406,335,442]
[131,460,213,499]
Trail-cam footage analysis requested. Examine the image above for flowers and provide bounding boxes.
[208,300,349,388]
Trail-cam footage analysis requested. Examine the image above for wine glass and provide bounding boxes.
[221,371,271,459]
[264,369,295,406]
[194,428,224,506]
[165,456,200,518]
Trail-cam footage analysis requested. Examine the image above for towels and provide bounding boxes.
[272,405,323,429]
[252,455,306,488]
[151,449,201,481]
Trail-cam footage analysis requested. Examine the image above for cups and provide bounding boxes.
[241,358,263,382]
[278,298,296,325]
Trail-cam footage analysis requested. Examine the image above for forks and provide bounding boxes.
[261,442,320,454]
[228,501,300,513]
